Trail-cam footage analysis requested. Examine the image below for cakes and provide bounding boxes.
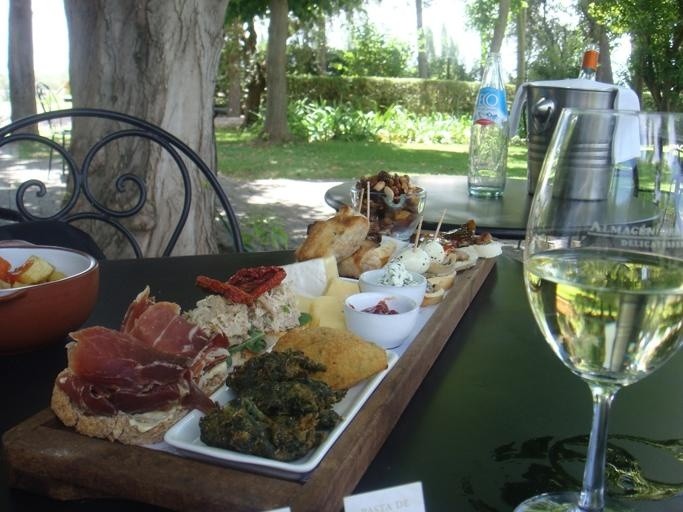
[398,220,502,308]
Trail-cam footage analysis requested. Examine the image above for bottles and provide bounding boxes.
[573,40,602,90]
[466,50,513,199]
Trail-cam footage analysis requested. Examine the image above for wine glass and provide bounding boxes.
[513,103,683,511]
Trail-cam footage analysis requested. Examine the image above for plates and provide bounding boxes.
[163,331,402,482]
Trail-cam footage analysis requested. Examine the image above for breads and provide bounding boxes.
[293,204,396,277]
[50,362,227,446]
[273,328,389,389]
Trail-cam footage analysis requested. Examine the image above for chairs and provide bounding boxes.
[1,107,245,255]
[35,81,72,175]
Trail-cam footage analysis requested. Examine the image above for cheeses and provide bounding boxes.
[299,276,361,330]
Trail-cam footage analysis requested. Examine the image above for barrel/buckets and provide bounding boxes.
[524,84,617,202]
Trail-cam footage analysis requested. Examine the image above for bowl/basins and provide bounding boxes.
[358,268,427,311]
[350,185,428,242]
[0,246,100,356]
[344,291,420,352]
